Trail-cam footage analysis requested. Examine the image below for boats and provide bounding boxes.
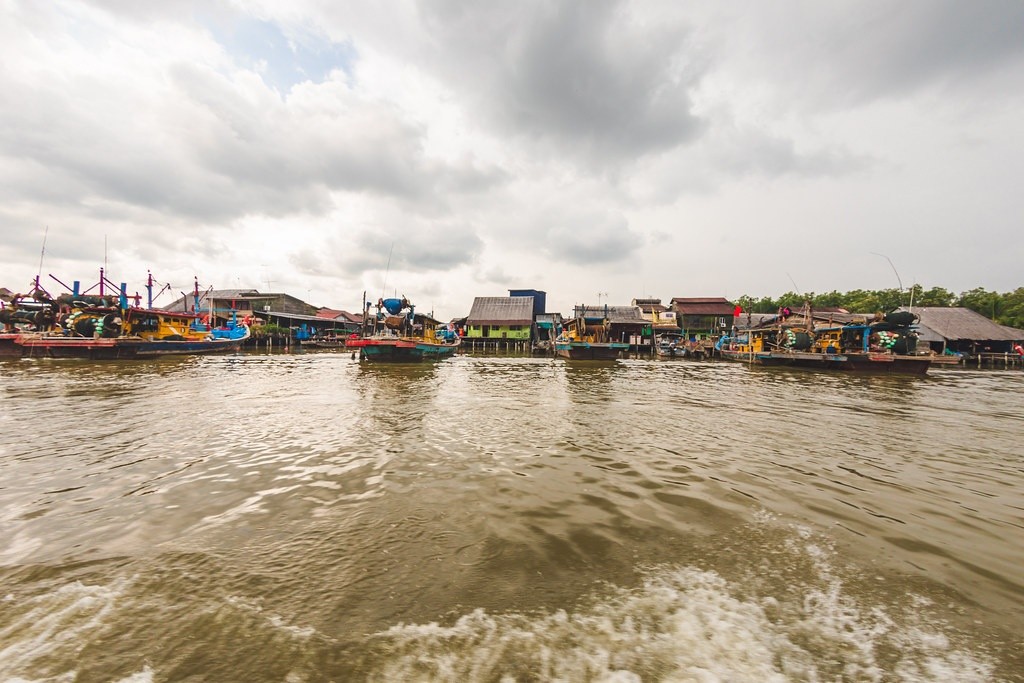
[720,296,937,376]
[344,290,463,367]
[648,295,715,359]
[548,301,631,360]
[0,226,252,357]
[298,334,343,348]
